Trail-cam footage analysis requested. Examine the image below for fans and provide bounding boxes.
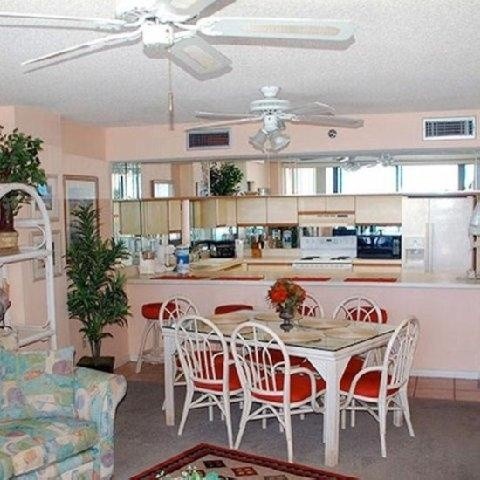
[185,87,367,155]
[2,1,356,113]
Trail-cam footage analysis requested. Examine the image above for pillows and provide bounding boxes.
[1,346,82,421]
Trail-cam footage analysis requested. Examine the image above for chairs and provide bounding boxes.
[330,294,383,328]
[320,314,421,458]
[228,320,329,465]
[174,314,268,450]
[157,296,218,423]
[272,293,326,320]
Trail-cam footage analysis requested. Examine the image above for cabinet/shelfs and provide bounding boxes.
[1,182,60,351]
[113,192,406,236]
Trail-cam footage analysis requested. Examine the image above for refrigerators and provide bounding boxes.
[400,195,477,280]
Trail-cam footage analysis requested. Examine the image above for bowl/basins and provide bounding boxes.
[257,187,269,196]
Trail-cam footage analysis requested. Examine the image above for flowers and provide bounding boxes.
[267,278,306,312]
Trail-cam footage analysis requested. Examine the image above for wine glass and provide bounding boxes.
[190,247,211,265]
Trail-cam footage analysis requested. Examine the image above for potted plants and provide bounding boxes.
[62,198,134,376]
[1,123,52,255]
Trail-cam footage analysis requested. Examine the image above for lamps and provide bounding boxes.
[248,128,291,154]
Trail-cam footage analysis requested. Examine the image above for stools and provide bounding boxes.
[135,302,181,373]
[213,303,256,315]
[344,304,388,323]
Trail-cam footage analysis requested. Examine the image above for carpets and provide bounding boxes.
[127,442,362,480]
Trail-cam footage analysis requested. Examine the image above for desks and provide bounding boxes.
[158,308,412,466]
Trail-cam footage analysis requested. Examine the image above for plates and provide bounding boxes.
[264,332,322,345]
[325,327,380,341]
[198,313,258,334]
[294,319,353,330]
[254,312,285,322]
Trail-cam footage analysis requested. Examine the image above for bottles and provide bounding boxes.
[133,236,189,277]
[235,224,298,258]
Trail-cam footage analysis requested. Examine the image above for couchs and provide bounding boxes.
[0,364,128,479]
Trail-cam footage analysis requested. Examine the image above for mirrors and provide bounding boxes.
[115,155,480,198]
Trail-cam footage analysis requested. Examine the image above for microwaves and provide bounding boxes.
[357,234,402,259]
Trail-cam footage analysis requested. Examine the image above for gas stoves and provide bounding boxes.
[292,255,358,265]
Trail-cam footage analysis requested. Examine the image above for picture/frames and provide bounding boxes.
[27,228,62,283]
[63,173,100,266]
[28,172,61,224]
